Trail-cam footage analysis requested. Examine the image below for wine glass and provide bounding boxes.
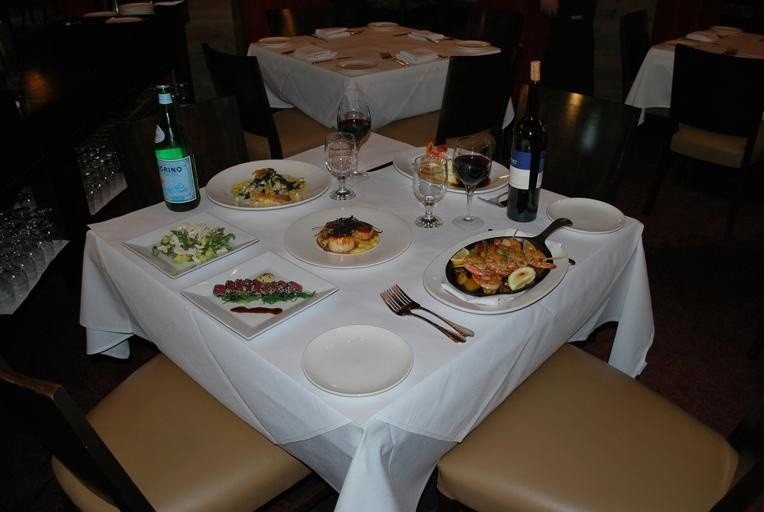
[452,136,492,231]
[1,199,63,309]
[325,133,358,200]
[337,100,372,182]
[74,145,124,213]
[412,155,448,231]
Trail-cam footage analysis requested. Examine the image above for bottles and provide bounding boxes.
[152,83,201,212]
[506,80,545,222]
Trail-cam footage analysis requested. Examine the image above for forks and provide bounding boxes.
[390,283,474,337]
[380,51,410,67]
[379,288,466,343]
[479,191,508,207]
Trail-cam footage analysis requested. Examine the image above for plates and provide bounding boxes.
[547,197,625,234]
[663,40,696,48]
[368,22,398,30]
[282,206,413,268]
[424,227,569,315]
[120,211,260,279]
[181,251,339,341]
[393,146,510,193]
[207,160,330,211]
[713,26,742,35]
[302,325,414,397]
[336,60,378,72]
[456,41,491,49]
[260,37,290,45]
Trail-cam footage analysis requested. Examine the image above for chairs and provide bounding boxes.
[665,43,764,228]
[198,46,284,160]
[433,344,752,511]
[515,85,642,206]
[434,52,519,144]
[1,353,312,512]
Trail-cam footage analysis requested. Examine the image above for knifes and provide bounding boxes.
[366,161,393,172]
